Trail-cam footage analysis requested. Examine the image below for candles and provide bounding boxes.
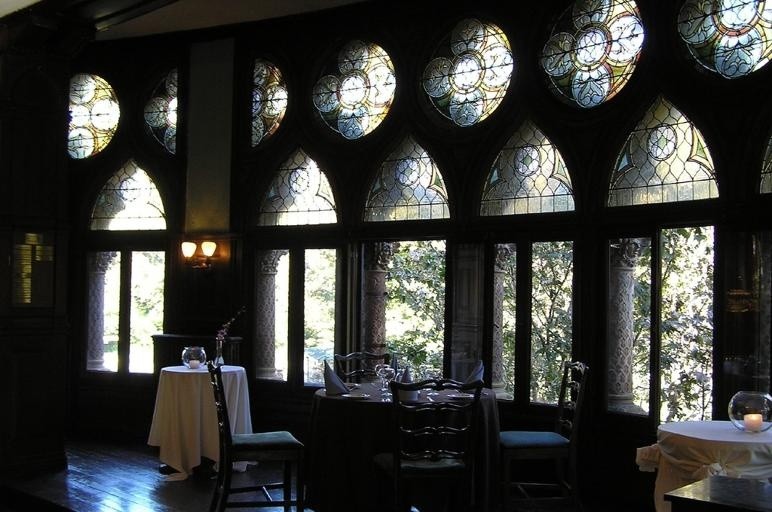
[744,413,763,430]
[190,360,200,368]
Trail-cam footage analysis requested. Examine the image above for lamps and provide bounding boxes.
[181,241,217,269]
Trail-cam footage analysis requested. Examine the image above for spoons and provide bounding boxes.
[350,384,361,391]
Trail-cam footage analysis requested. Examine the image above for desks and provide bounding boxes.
[309,383,501,511]
[636,419,772,512]
[147,365,257,482]
[664,477,772,512]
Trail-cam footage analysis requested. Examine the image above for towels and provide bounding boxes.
[464,359,484,384]
[401,366,417,401]
[391,353,401,372]
[323,360,350,395]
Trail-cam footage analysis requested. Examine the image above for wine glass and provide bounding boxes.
[375,363,395,402]
[418,364,441,396]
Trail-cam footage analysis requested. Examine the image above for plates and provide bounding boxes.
[446,393,474,399]
[344,383,362,387]
[342,393,370,398]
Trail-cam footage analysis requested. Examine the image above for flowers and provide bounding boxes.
[217,304,248,359]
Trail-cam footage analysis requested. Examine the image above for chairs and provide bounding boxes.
[498,360,590,512]
[334,351,390,384]
[389,379,484,512]
[207,361,305,512]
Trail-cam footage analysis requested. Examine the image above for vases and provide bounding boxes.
[215,340,225,366]
